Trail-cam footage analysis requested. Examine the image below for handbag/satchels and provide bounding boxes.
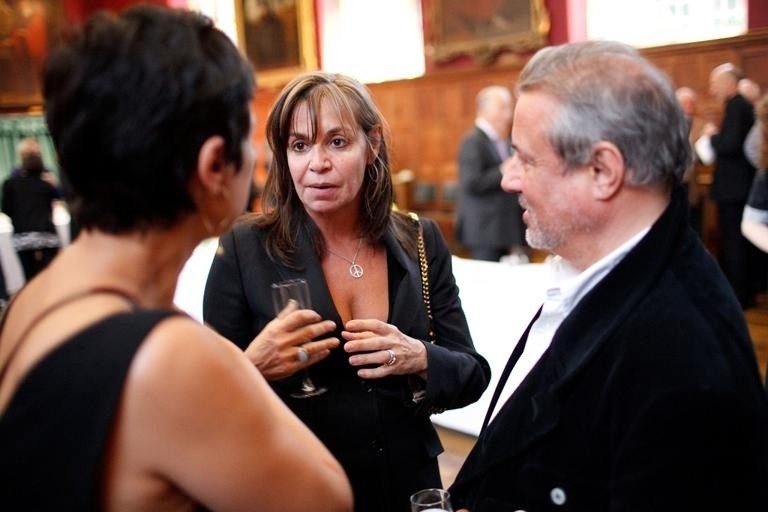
[404,211,439,345]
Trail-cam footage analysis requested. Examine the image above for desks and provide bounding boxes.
[410,258,551,442]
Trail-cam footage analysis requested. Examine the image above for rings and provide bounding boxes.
[387,348,397,366]
[294,344,310,365]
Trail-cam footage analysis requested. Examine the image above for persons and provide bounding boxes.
[1,136,67,301]
[245,140,277,214]
[452,86,535,263]
[439,40,768,512]
[202,76,495,511]
[1,5,357,510]
[674,60,768,312]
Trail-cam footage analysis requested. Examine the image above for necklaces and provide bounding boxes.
[326,238,364,278]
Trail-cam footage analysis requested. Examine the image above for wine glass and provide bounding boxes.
[271,277,327,401]
[408,486,455,512]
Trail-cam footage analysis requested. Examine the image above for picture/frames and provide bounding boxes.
[419,1,555,69]
[233,1,322,91]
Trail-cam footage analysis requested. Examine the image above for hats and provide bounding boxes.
[21,155,51,172]
